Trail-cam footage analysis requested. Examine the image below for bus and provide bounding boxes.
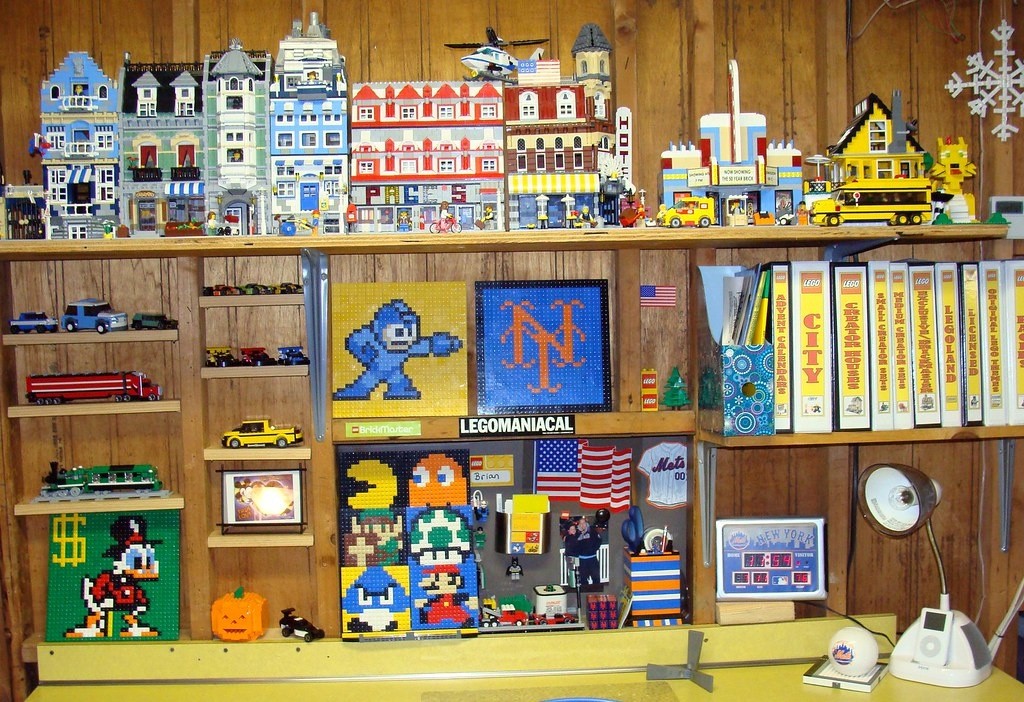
[810,174,934,226]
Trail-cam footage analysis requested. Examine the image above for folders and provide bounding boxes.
[753,258,1024,434]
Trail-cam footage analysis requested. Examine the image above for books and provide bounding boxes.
[722,262,771,397]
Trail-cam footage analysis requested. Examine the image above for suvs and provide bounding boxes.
[61,296,128,335]
[132,312,180,330]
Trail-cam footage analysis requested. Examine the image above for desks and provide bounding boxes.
[22,611,1024,702]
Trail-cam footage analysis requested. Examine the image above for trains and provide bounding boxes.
[38,458,164,498]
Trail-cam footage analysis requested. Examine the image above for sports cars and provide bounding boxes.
[532,611,575,625]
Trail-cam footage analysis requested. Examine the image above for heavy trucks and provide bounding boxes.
[24,369,163,406]
[481,606,527,627]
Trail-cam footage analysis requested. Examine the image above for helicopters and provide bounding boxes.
[443,27,551,85]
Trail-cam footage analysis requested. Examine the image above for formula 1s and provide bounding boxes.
[278,607,326,642]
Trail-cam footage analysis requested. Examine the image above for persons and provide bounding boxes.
[309,71,316,80]
[71,85,88,106]
[312,210,320,237]
[579,201,695,228]
[397,208,412,231]
[207,211,220,236]
[440,201,452,233]
[485,205,495,229]
[796,201,809,226]
[231,149,242,162]
[731,199,744,215]
[565,517,602,585]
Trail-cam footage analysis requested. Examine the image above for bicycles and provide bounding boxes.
[430,215,462,233]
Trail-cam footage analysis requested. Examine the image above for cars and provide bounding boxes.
[204,344,310,368]
[201,281,304,296]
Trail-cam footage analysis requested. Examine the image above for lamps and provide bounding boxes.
[857,463,992,689]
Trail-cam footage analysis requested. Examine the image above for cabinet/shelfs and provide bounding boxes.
[0,224,1024,701]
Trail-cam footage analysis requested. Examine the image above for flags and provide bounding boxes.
[535,439,632,513]
[640,285,676,308]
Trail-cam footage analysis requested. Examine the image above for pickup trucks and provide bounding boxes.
[8,311,61,334]
[221,418,304,448]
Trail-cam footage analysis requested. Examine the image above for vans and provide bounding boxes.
[657,196,717,227]
